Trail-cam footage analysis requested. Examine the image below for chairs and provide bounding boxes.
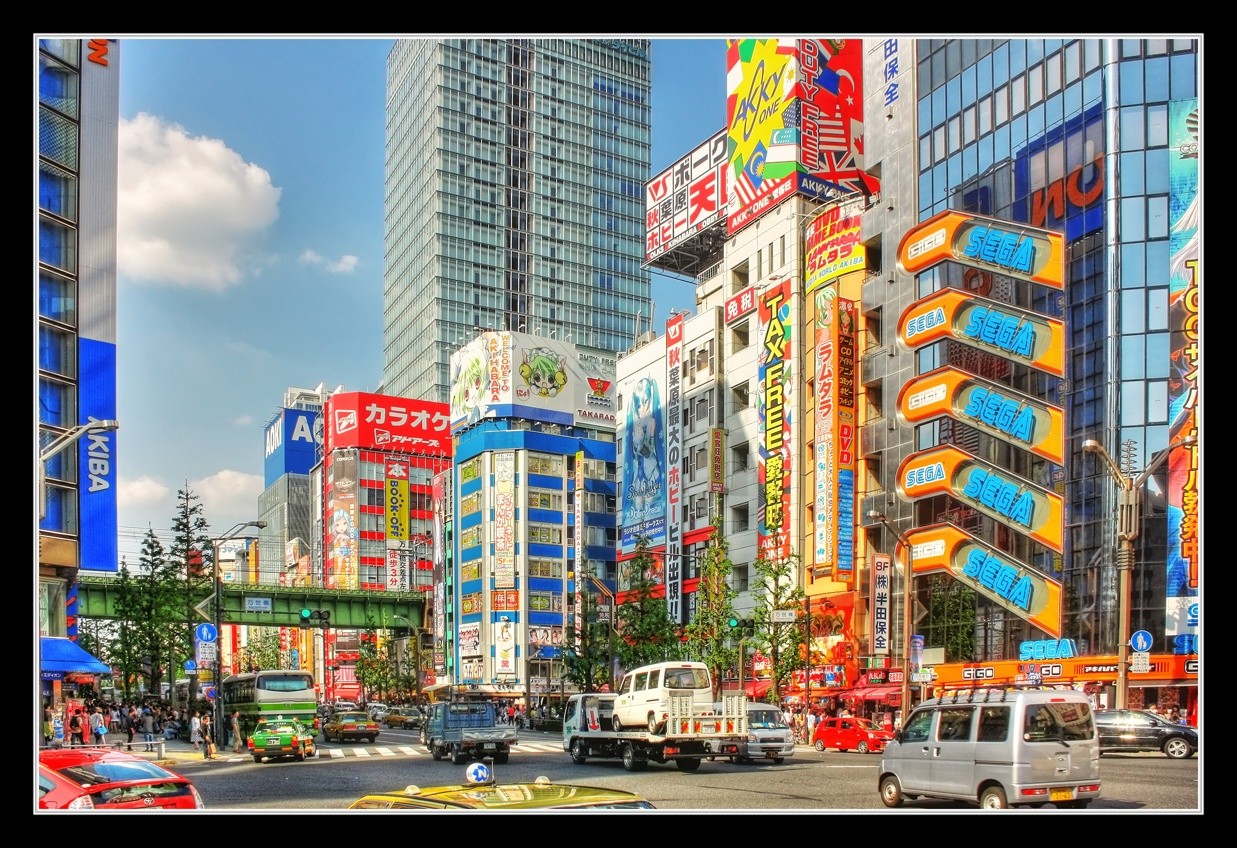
[642,676,680,688]
[946,713,968,739]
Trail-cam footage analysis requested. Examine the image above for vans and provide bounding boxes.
[703,702,795,765]
[612,661,713,735]
[877,683,1102,812]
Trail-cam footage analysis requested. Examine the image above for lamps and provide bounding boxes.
[442,322,574,353]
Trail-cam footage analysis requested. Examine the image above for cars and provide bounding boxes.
[318,702,426,745]
[812,718,894,754]
[419,720,427,745]
[39,748,205,809]
[248,718,317,763]
[348,775,657,809]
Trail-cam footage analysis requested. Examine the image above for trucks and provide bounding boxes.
[559,690,750,774]
[426,701,517,766]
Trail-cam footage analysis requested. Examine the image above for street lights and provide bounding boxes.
[40,419,120,521]
[393,614,421,709]
[580,571,615,693]
[215,520,268,752]
[865,510,914,730]
[1081,435,1201,710]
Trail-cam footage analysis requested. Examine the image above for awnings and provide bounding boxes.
[713,681,773,698]
[839,687,902,700]
[784,690,846,698]
[39,636,111,680]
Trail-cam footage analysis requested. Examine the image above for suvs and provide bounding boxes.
[1093,708,1198,760]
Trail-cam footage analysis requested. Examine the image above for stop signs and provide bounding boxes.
[314,683,320,693]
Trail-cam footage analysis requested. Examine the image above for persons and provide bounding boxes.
[43,691,241,760]
[783,705,852,747]
[459,624,510,650]
[494,702,544,731]
[360,703,377,718]
[529,629,562,645]
[1149,703,1183,724]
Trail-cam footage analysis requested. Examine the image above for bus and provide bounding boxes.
[222,669,319,739]
[164,680,217,717]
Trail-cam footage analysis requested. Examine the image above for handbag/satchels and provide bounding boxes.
[207,740,217,754]
[95,726,107,735]
[229,734,244,747]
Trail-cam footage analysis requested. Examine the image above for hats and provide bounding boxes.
[144,708,150,713]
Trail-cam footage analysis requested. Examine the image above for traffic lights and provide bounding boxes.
[320,619,331,629]
[744,628,754,637]
[299,608,331,621]
[727,618,754,628]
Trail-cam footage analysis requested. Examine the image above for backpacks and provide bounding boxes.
[69,716,81,730]
